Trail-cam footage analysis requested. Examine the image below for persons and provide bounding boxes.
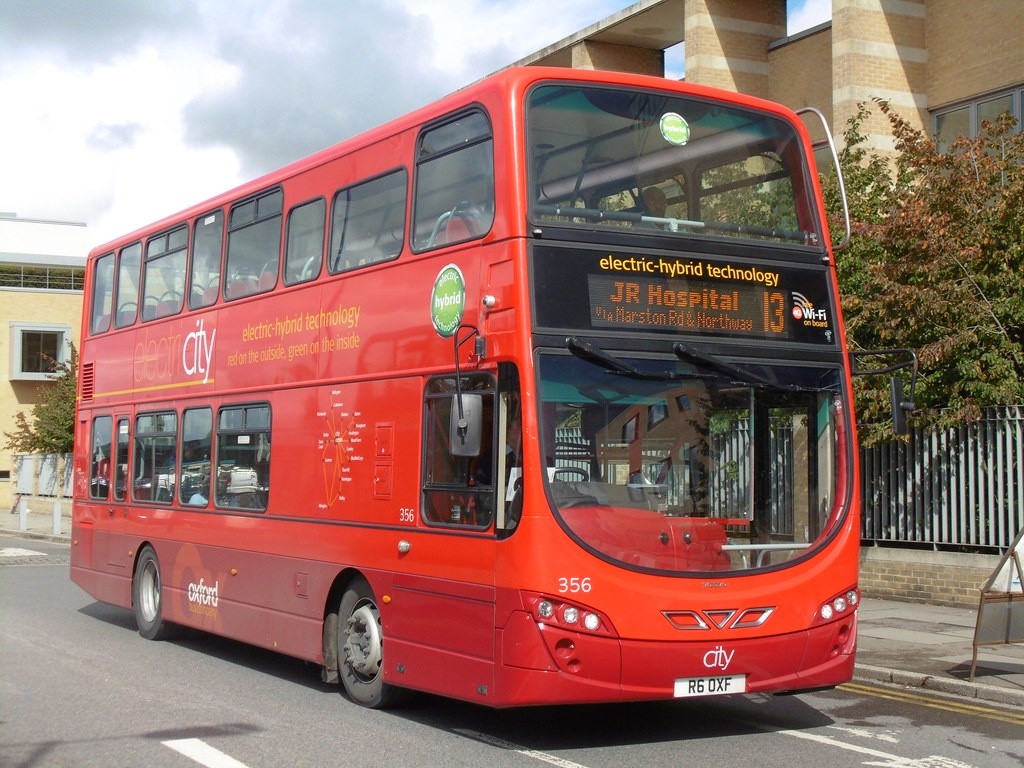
[158,436,265,507]
[632,187,686,232]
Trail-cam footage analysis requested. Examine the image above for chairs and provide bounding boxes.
[100,211,485,330]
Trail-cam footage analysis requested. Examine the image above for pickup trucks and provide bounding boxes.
[144,459,259,502]
[88,462,152,494]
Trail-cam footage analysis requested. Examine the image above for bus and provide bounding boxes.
[70,61,924,711]
